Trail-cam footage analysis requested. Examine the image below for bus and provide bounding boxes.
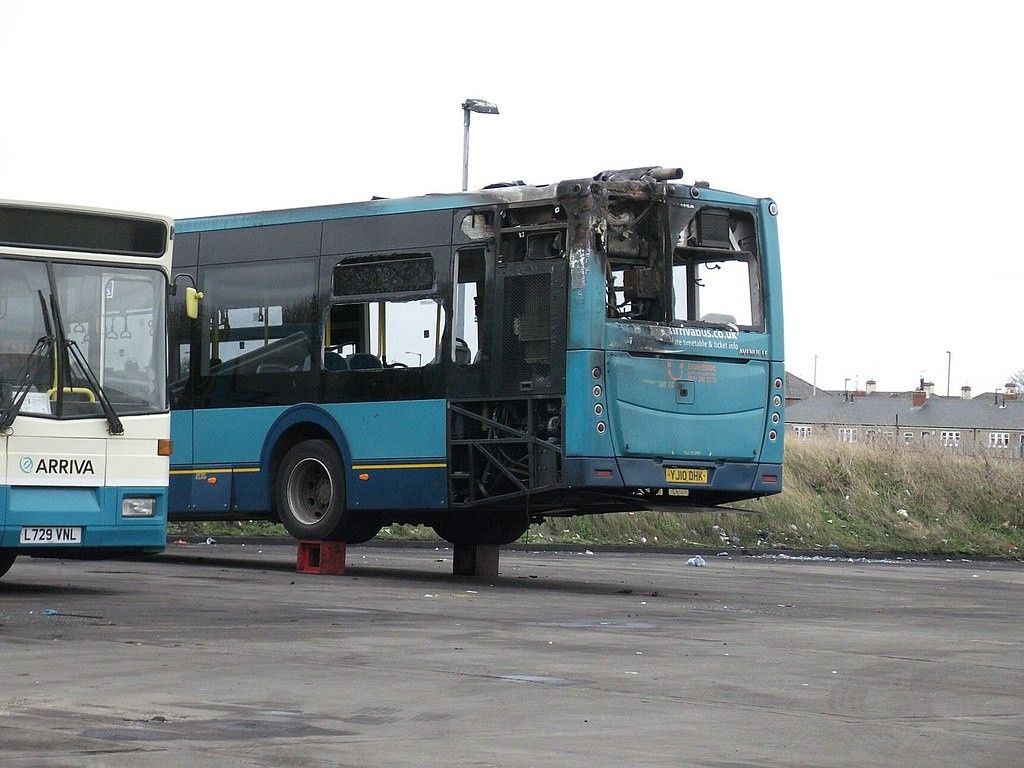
[65,166,784,546]
[1,200,203,578]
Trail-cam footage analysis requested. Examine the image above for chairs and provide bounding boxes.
[256,337,482,374]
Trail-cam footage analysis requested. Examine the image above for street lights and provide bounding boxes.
[405,352,421,367]
[946,351,951,397]
[458,98,502,338]
[844,379,851,391]
